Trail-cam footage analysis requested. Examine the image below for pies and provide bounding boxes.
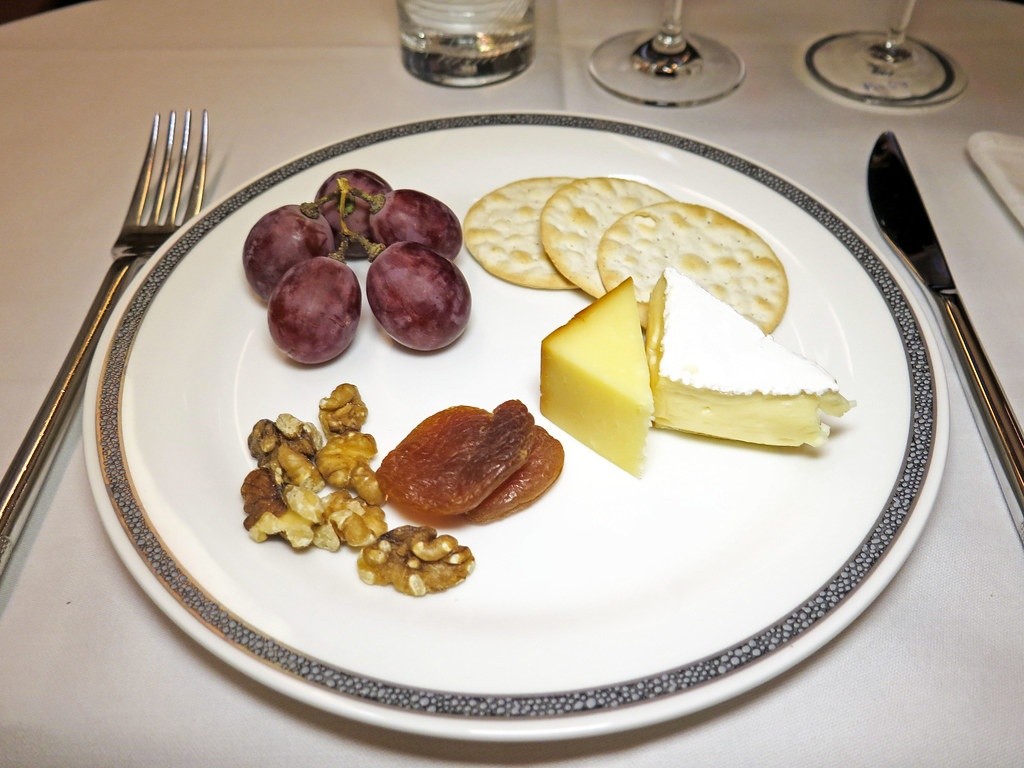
[465,175,789,338]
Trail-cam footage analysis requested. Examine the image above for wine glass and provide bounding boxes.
[586,0,746,105]
[793,1,967,114]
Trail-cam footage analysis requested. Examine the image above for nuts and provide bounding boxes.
[241,382,565,597]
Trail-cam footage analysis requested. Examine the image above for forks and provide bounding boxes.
[2,108,208,575]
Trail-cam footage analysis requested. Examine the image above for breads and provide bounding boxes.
[540,267,849,481]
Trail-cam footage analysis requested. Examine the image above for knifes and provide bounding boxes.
[867,129,1024,549]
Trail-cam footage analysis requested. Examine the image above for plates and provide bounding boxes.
[967,131,1023,228]
[81,113,949,744]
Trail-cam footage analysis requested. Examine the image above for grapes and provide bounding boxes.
[241,169,473,365]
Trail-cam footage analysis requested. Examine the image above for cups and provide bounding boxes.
[396,1,535,88]
